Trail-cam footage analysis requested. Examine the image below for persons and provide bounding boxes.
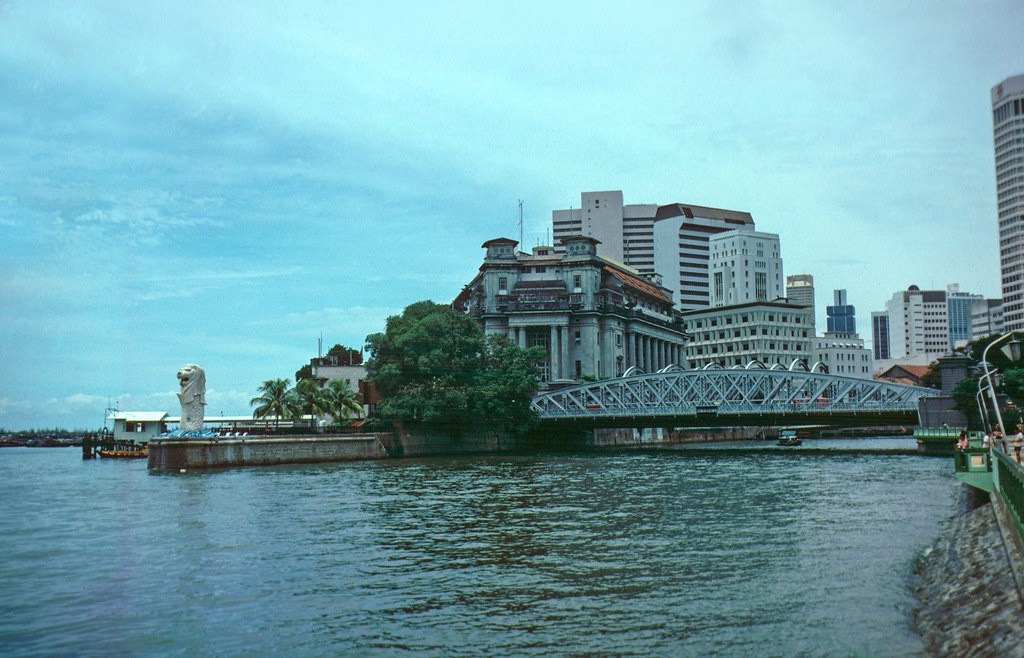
[816,399,822,408]
[791,400,800,410]
[229,423,233,435]
[983,431,991,447]
[992,423,1002,438]
[1009,420,1024,466]
[265,422,276,434]
[958,430,969,450]
[942,421,949,433]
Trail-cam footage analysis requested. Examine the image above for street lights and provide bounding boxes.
[982,329,1024,454]
[977,385,992,434]
[978,368,1000,434]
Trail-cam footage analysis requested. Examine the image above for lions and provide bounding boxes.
[176,364,207,432]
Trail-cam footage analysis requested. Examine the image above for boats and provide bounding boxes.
[777,428,803,447]
[96,449,148,458]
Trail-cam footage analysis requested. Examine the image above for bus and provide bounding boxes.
[791,398,828,410]
